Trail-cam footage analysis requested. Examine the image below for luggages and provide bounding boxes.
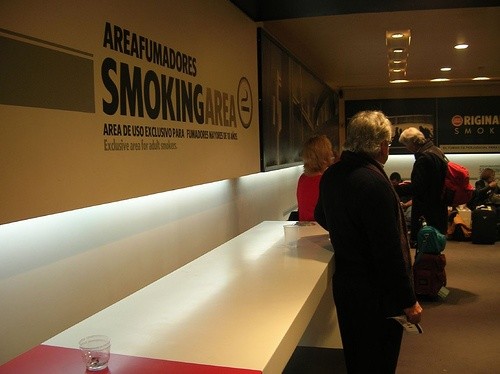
[413,253,448,304]
[472,203,496,244]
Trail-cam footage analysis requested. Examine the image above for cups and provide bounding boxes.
[77,334,110,371]
[284,224,298,248]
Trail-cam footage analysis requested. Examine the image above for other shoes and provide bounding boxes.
[408,240,418,248]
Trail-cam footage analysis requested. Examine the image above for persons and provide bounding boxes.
[296,134,334,222]
[399,128,449,301]
[314,110,422,374]
[471,167,500,213]
[390,172,413,224]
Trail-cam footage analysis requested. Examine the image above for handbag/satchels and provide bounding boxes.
[418,226,446,255]
[436,147,469,205]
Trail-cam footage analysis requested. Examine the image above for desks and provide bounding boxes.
[0,220,343,374]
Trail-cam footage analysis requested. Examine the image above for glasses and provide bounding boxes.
[386,139,393,147]
